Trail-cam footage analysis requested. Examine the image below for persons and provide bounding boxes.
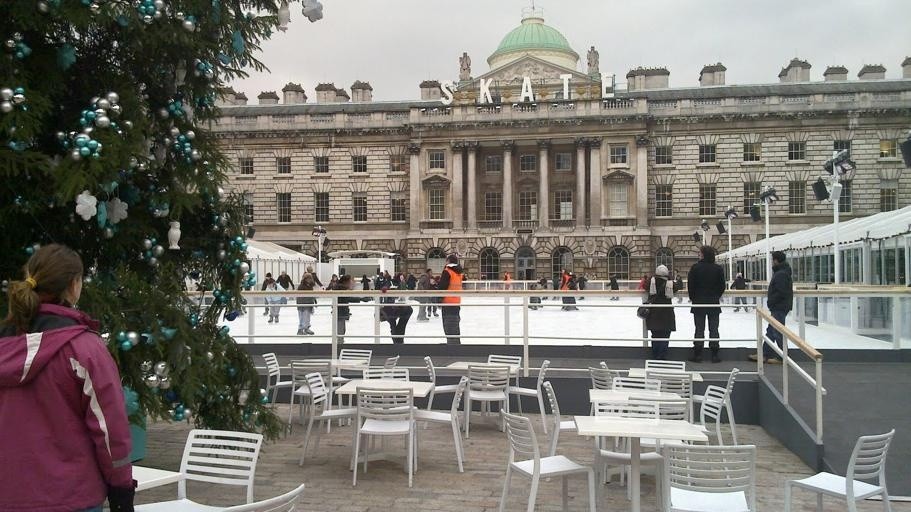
[296,256,467,345]
[687,245,726,363]
[503,271,511,290]
[276,271,295,304]
[265,278,287,322]
[261,273,276,316]
[0,242,138,512]
[747,251,793,365]
[729,272,753,312]
[637,265,684,368]
[528,268,588,312]
[606,274,619,301]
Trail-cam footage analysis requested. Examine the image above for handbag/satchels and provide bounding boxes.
[637,302,651,318]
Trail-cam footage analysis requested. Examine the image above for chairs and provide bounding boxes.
[353,385,416,489]
[424,355,467,430]
[406,376,469,473]
[592,397,665,509]
[499,408,598,511]
[133,428,265,510]
[298,372,358,471]
[539,382,578,484]
[222,481,306,512]
[583,355,744,488]
[780,428,896,512]
[463,364,512,438]
[500,359,552,435]
[661,442,760,510]
[480,354,523,418]
[254,351,412,431]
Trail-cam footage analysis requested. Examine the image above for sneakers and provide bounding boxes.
[768,356,782,364]
[749,353,766,362]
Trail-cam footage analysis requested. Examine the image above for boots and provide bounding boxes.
[275,316,278,322]
[297,329,306,335]
[305,326,314,334]
[268,315,273,322]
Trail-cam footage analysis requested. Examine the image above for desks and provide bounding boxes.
[333,378,435,467]
[134,463,186,493]
[573,415,708,512]
[446,359,520,426]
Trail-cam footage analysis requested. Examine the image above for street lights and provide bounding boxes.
[898,133,911,168]
[694,219,710,250]
[312,226,329,285]
[812,145,858,284]
[750,184,779,284]
[716,207,738,290]
[237,220,256,239]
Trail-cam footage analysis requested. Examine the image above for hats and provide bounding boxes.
[656,264,669,276]
